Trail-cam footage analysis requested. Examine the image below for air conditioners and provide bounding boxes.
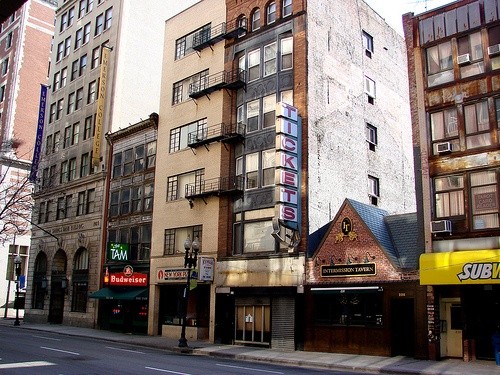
[457,53,472,66]
[488,44,500,56]
[430,220,452,234]
[437,142,453,153]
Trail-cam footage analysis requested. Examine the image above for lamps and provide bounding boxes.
[347,254,357,264]
[42,276,49,295]
[61,277,69,295]
[364,252,376,263]
[330,255,342,266]
[314,257,325,267]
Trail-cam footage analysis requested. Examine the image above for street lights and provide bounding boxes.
[177,240,200,348]
[13,255,22,326]
